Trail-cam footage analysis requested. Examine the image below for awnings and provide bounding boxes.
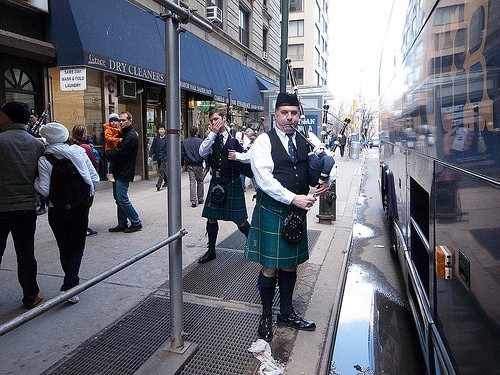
[48,0,265,112]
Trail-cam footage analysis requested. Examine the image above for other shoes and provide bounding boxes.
[124,224,142,233]
[109,224,128,232]
[86,229,97,236]
[156,184,167,190]
[191,200,203,207]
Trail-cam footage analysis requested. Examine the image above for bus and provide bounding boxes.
[373,0,500,375]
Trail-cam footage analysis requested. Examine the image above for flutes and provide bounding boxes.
[223,87,264,184]
[286,57,352,219]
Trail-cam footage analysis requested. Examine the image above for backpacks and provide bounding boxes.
[44,154,90,218]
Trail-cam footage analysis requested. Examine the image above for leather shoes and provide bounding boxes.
[258,314,273,342]
[277,312,316,330]
[198,251,216,263]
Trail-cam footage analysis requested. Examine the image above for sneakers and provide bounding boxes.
[25,291,43,309]
[60,292,79,303]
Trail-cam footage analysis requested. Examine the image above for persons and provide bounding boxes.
[67,120,100,237]
[0,101,46,310]
[31,113,45,139]
[247,91,337,342]
[102,109,143,236]
[197,109,251,267]
[34,117,100,302]
[84,134,100,164]
[226,119,262,191]
[104,113,139,184]
[179,125,206,209]
[146,123,170,190]
[322,129,371,156]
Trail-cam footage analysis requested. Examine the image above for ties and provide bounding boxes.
[220,135,224,150]
[286,133,298,164]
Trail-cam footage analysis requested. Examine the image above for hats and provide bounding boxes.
[109,114,119,122]
[1,101,30,124]
[39,123,69,143]
[86,136,93,143]
[276,93,299,106]
[31,107,36,115]
